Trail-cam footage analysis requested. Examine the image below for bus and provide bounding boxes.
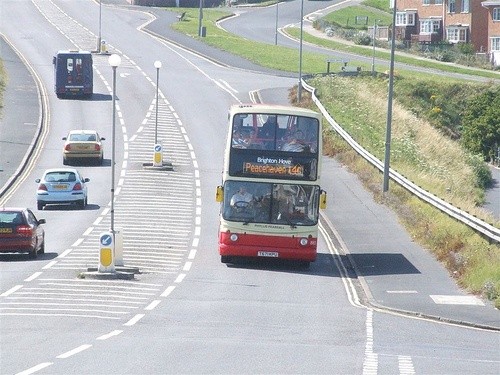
[215,104,327,267]
[52,48,93,99]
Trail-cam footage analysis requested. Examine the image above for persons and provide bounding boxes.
[232,125,317,151]
[230,185,253,211]
[297,191,310,203]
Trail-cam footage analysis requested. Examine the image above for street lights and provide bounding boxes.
[107,53,121,264]
[154,60,162,144]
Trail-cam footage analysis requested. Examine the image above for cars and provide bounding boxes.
[62,129,106,165]
[34,167,90,210]
[0,207,46,259]
